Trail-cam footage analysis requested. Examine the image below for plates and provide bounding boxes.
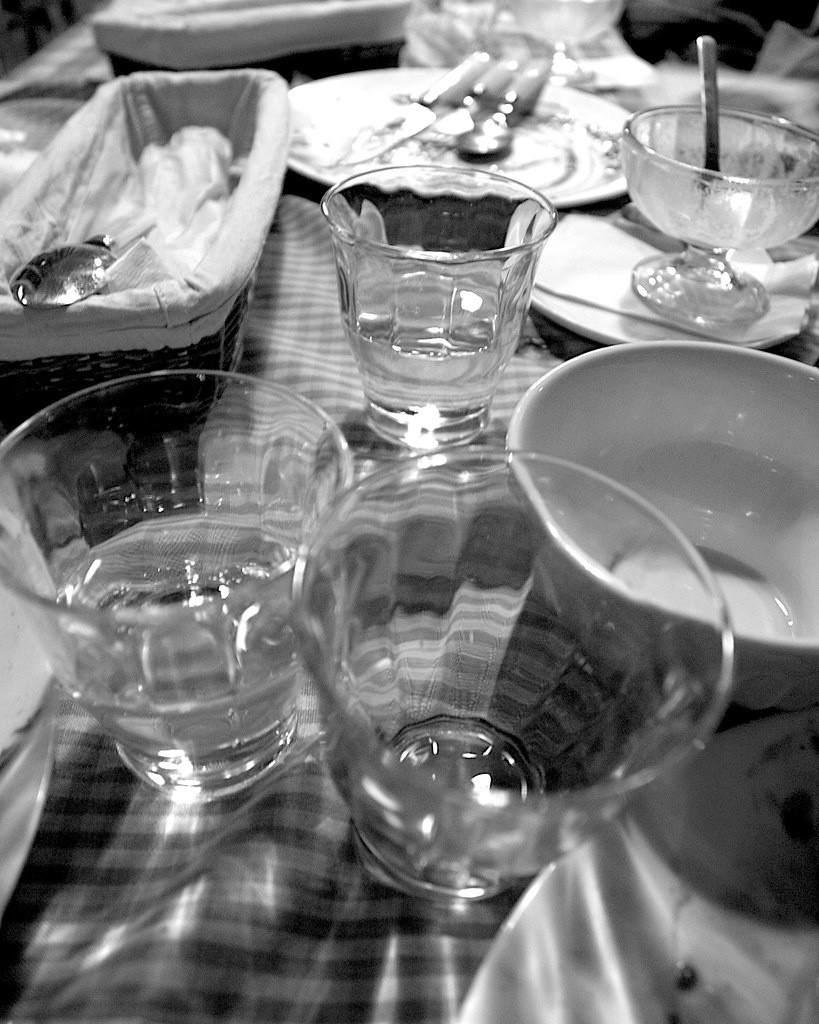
[288,67,634,209]
[455,710,819,1024]
[503,199,810,346]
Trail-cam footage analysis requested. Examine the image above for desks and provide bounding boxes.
[0,0,819,1024]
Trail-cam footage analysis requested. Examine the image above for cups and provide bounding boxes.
[0,367,355,806]
[289,447,735,906]
[319,164,558,448]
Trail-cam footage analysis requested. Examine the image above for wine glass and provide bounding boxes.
[619,105,819,326]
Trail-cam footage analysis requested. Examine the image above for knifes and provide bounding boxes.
[340,50,490,165]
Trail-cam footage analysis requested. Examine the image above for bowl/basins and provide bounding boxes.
[504,339,818,712]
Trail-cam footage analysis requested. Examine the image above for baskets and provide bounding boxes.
[0,68,294,429]
[89,0,411,87]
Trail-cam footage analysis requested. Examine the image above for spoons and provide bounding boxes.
[457,118,512,155]
[9,243,118,310]
